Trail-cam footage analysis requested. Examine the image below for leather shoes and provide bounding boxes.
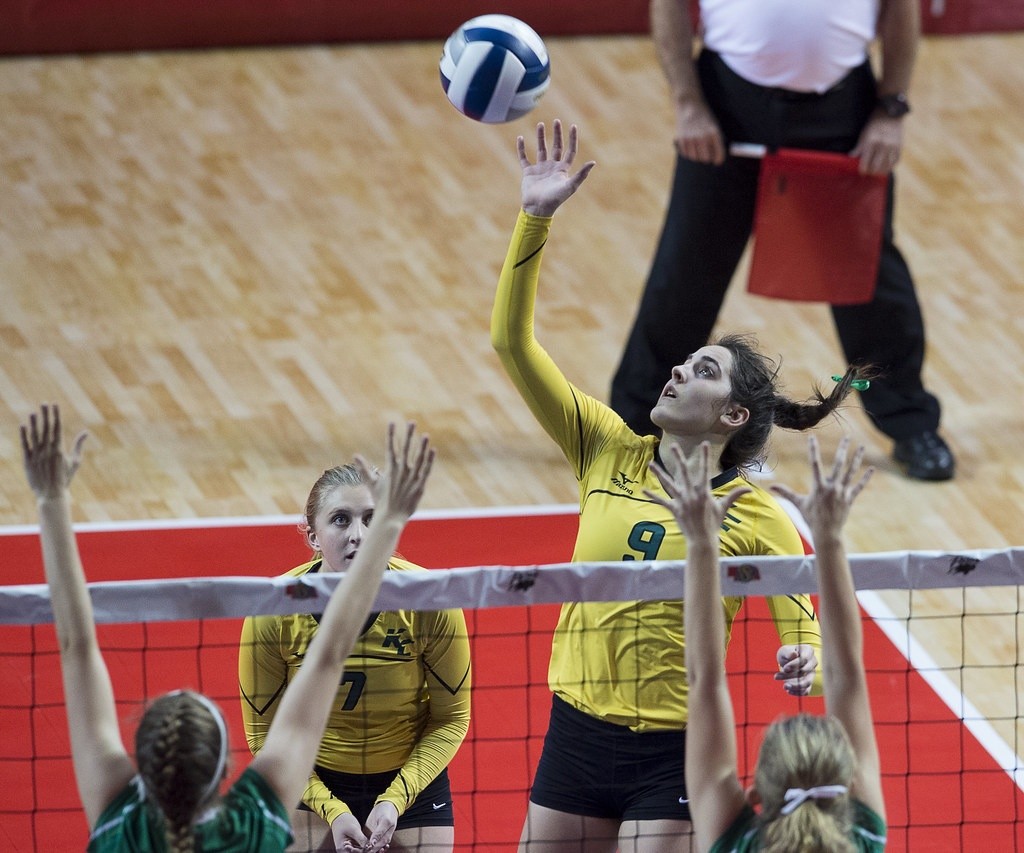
[894,431,954,481]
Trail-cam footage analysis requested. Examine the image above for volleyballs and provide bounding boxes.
[437,13,552,126]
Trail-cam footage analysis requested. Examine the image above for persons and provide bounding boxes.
[20,401,437,853]
[238,465,472,853]
[608,0,959,482]
[642,435,889,852]
[490,117,827,853]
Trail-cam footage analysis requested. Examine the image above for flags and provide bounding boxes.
[746,147,888,304]
